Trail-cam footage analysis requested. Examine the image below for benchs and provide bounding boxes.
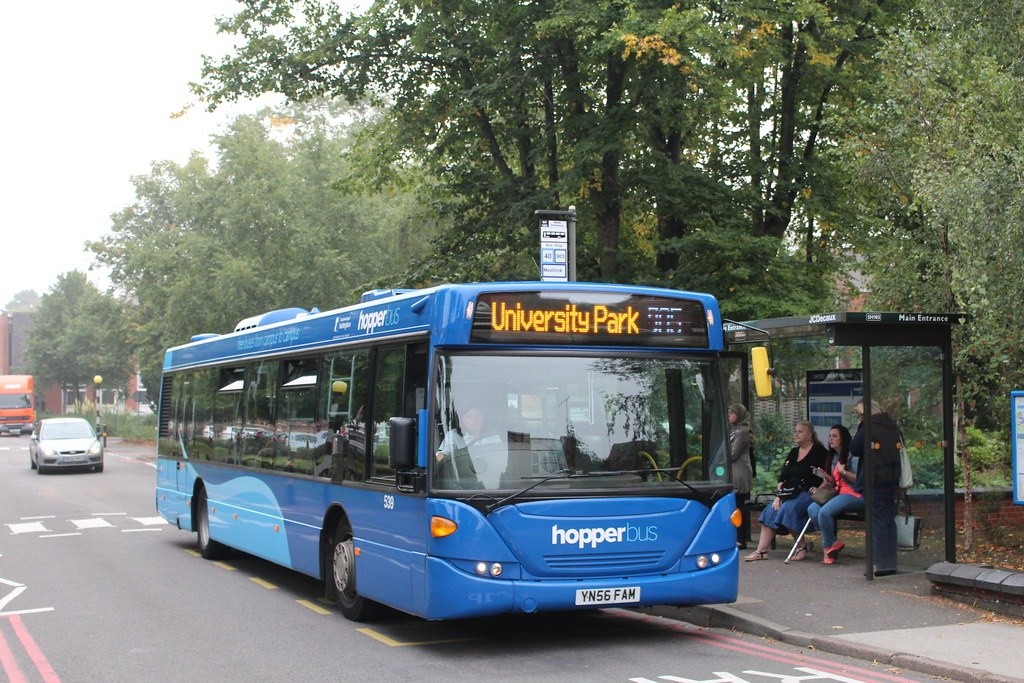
[744,492,864,558]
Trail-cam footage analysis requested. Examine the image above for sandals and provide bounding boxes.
[791,543,807,561]
[744,551,768,560]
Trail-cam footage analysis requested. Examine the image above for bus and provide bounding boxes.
[151,281,777,623]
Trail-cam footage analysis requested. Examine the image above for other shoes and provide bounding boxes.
[827,541,845,557]
[824,558,837,563]
[738,544,747,550]
[863,570,897,576]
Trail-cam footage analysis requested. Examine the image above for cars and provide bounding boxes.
[29,418,104,474]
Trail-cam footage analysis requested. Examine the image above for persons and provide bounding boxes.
[708,403,754,550]
[850,398,905,576]
[817,424,864,564]
[744,420,829,561]
[435,402,502,462]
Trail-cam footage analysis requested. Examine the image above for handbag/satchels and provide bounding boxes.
[898,432,913,487]
[777,486,798,499]
[750,448,756,478]
[895,489,921,547]
[811,482,838,505]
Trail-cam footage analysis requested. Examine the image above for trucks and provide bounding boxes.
[0,375,35,436]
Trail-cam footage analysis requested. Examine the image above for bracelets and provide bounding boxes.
[843,471,846,476]
